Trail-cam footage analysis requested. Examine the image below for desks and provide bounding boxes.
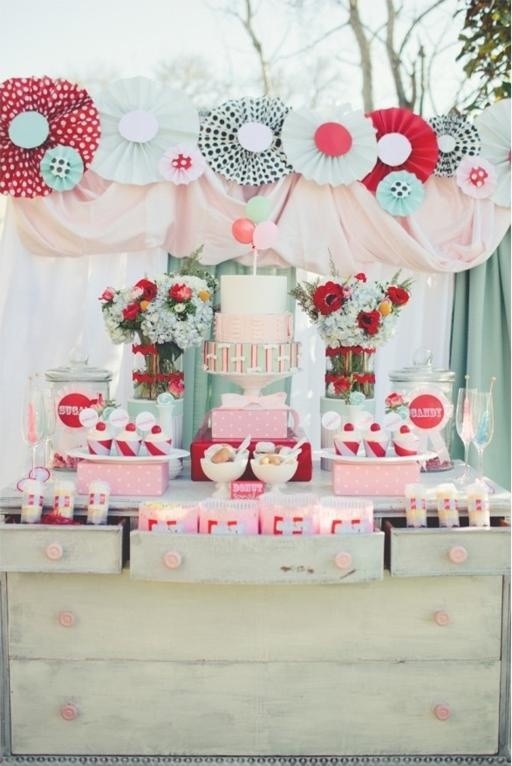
[0,459,512,761]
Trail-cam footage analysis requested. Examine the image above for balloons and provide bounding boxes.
[246,196,271,225]
[252,222,278,250]
[232,219,256,243]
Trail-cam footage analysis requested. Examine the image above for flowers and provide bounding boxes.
[288,267,413,397]
[96,246,220,399]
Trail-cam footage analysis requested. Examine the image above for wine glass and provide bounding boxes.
[456,387,495,492]
[19,396,53,478]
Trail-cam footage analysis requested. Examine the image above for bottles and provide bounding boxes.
[47,349,111,472]
[388,346,456,472]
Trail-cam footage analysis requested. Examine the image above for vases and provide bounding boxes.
[324,341,378,398]
[128,342,184,399]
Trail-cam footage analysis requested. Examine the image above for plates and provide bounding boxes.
[319,448,439,462]
[69,447,190,465]
